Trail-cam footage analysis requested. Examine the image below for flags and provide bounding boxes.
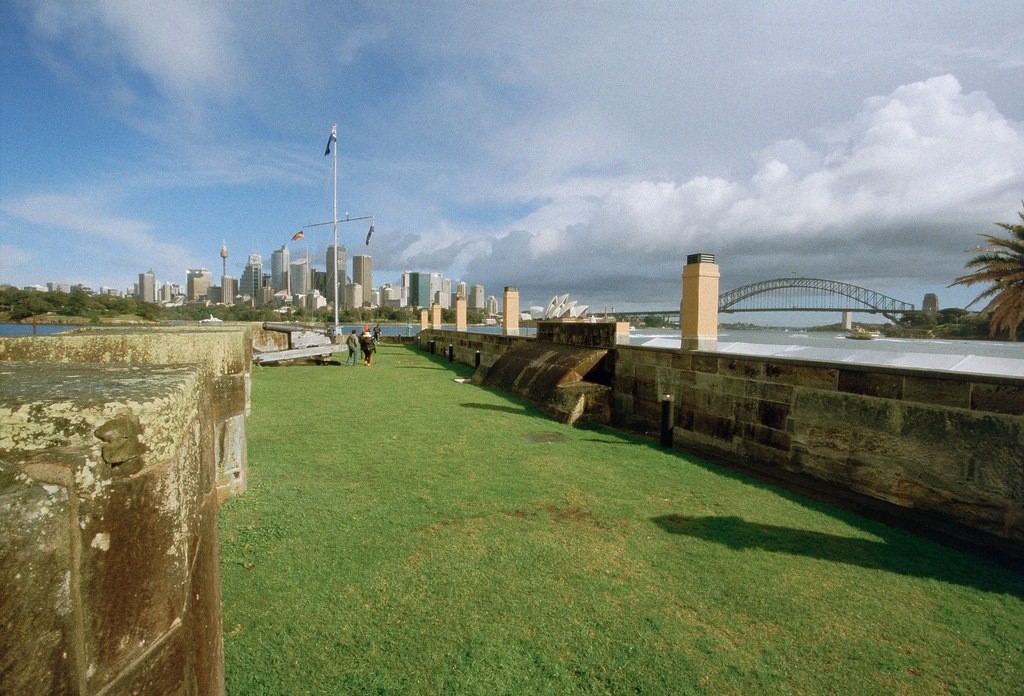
[325,127,337,157]
[290,230,304,241]
[366,219,375,245]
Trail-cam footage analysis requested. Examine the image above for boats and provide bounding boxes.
[844,325,880,341]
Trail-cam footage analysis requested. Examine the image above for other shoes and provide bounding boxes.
[364,361,367,366]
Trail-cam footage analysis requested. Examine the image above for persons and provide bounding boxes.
[373,324,381,344]
[346,330,360,368]
[359,325,377,367]
